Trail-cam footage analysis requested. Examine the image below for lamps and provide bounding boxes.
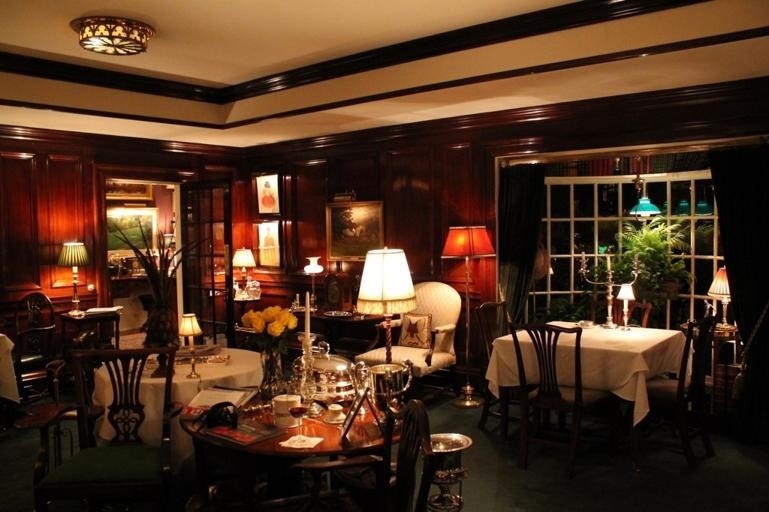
[629,180,661,218]
[57,242,90,317]
[70,15,153,56]
[707,266,738,339]
[355,248,419,363]
[440,226,496,409]
[303,257,324,309]
[231,246,261,302]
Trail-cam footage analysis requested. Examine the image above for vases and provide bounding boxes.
[161,233,175,248]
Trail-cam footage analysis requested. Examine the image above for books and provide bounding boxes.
[178,382,258,421]
[202,418,290,446]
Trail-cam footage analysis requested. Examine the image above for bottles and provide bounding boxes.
[233,276,261,300]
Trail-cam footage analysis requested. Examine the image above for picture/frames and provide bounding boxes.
[105,181,153,201]
[104,208,159,258]
[255,174,283,268]
[325,200,384,262]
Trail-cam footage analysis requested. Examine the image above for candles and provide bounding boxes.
[580,251,641,272]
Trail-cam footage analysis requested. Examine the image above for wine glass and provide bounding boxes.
[347,366,370,414]
[288,393,309,443]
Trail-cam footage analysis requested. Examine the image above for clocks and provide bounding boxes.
[323,272,354,312]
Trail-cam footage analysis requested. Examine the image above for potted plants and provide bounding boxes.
[114,216,212,349]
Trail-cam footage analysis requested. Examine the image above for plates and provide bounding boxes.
[322,415,347,425]
[323,311,353,317]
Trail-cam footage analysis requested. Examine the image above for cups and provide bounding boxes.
[274,395,302,428]
[328,403,343,419]
[202,334,228,359]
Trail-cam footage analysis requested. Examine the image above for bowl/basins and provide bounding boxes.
[577,320,594,329]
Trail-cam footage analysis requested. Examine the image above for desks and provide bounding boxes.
[681,322,740,423]
